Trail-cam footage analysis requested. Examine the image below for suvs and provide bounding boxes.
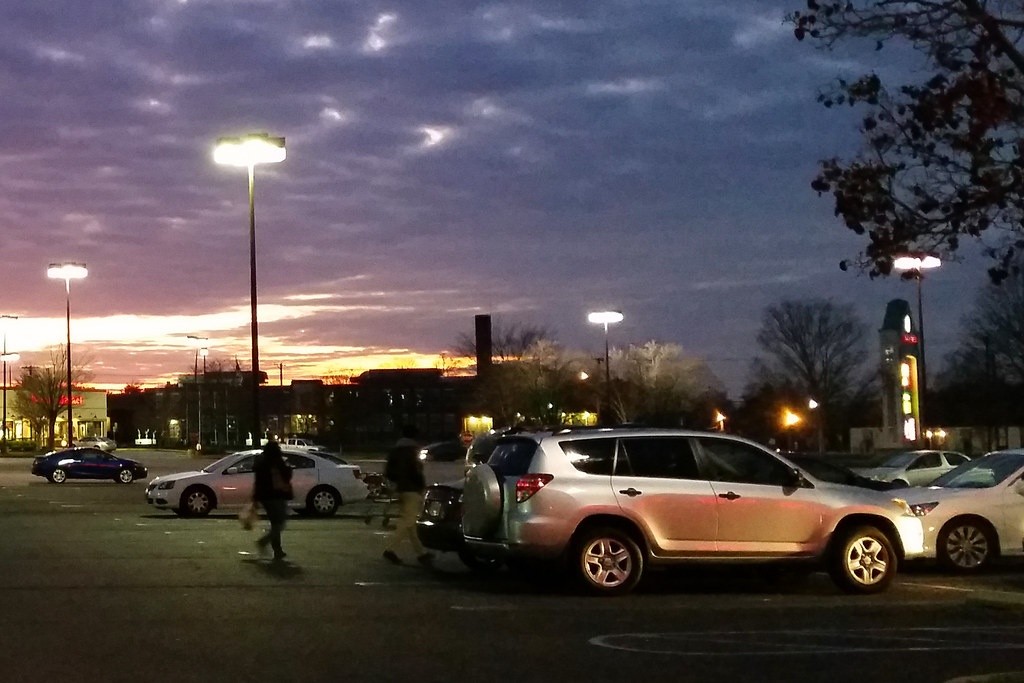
[462,424,923,599]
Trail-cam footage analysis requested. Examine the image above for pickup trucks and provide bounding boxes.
[279,439,326,452]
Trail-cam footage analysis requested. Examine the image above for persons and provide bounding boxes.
[382,427,438,566]
[252,442,294,561]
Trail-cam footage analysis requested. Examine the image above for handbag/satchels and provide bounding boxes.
[272,462,294,502]
[238,505,260,529]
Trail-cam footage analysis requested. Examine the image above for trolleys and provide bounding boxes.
[361,471,406,528]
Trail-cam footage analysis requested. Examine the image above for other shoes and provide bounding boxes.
[253,542,285,559]
[382,550,402,565]
[417,553,436,564]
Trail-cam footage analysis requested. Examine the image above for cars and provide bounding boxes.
[417,441,468,486]
[856,449,994,487]
[67,436,116,453]
[416,479,510,573]
[31,447,147,484]
[146,450,369,521]
[464,428,507,473]
[880,448,1024,576]
[745,455,904,492]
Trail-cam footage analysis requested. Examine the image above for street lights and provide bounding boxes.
[893,251,941,450]
[47,262,88,447]
[217,132,286,450]
[0,315,18,442]
[0,352,20,387]
[187,336,208,382]
[200,348,208,374]
[586,310,624,428]
